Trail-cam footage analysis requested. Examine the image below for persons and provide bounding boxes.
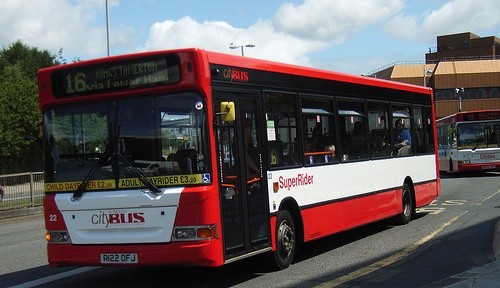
[489,126,500,144]
[389,117,412,157]
[128,116,392,188]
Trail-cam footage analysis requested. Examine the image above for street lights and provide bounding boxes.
[423,65,433,87]
[230,44,255,56]
[454,87,464,112]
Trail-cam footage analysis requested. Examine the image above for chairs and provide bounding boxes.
[157,149,197,175]
[268,129,425,167]
[458,138,484,148]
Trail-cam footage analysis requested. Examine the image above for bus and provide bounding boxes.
[419,110,500,176]
[37,48,440,270]
